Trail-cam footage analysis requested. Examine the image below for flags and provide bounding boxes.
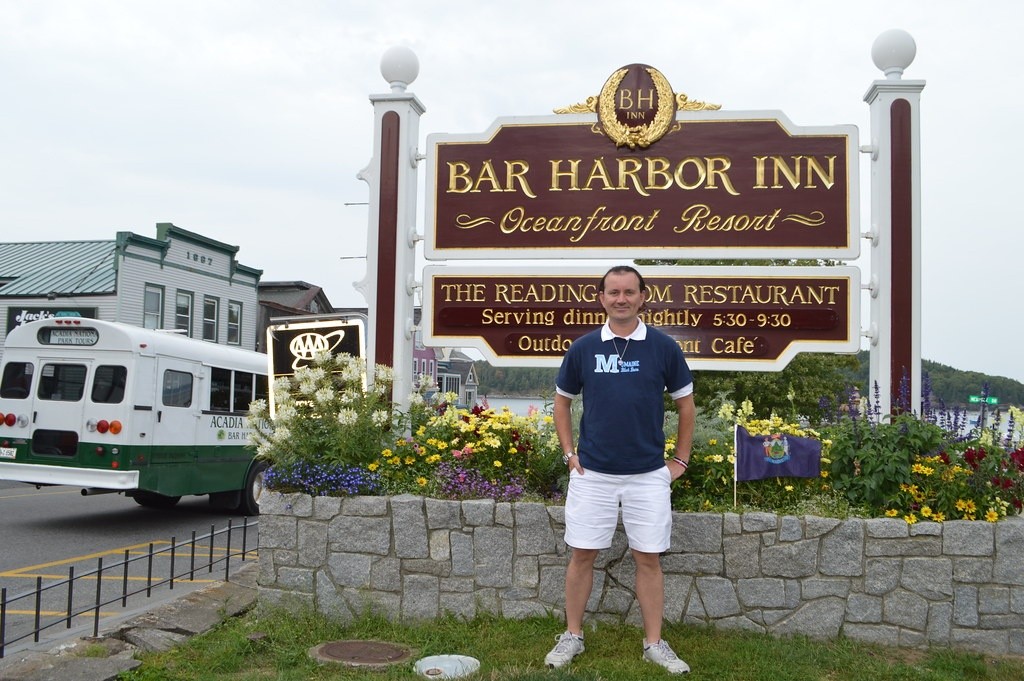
[737,425,821,480]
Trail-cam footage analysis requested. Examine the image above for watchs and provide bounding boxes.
[562,452,575,465]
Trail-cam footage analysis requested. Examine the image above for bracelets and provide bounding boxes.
[673,457,688,469]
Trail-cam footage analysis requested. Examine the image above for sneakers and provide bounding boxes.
[642,638,691,676]
[544,628,585,669]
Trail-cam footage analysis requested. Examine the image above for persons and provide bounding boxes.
[544,264,695,674]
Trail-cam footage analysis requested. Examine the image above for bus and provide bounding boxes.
[1,314,396,516]
